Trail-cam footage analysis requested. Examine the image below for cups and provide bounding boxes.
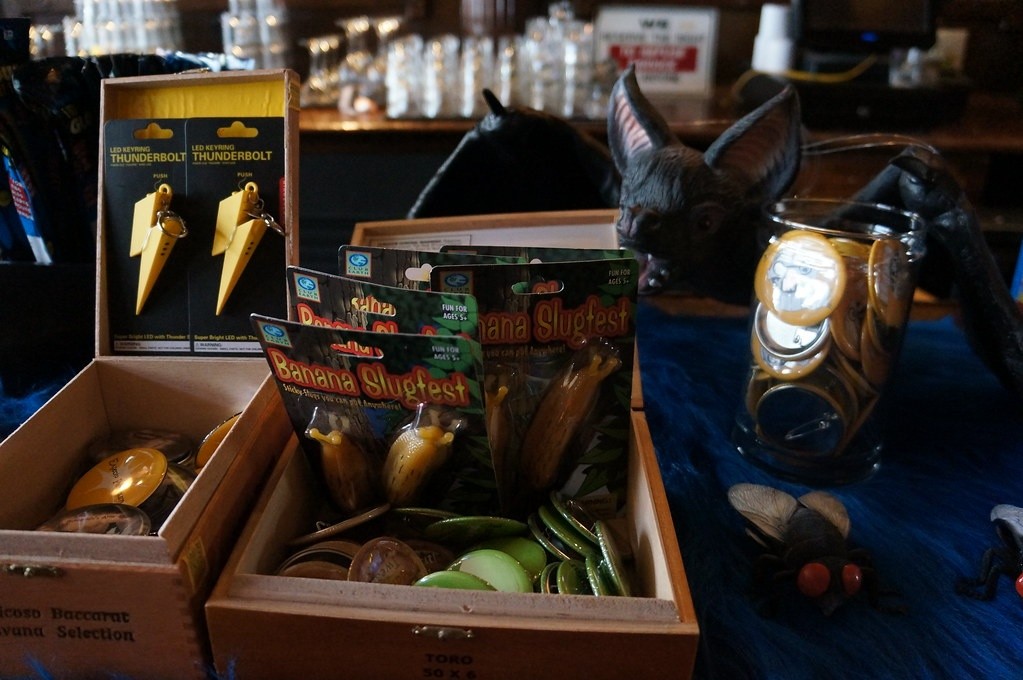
[385,2,611,120]
[730,196,927,487]
[29,1,288,70]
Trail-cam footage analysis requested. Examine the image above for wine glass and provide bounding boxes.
[297,16,404,115]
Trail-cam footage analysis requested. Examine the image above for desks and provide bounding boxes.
[2,282,1023,680]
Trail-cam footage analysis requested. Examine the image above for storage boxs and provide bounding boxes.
[1,69,301,680]
[205,208,703,680]
